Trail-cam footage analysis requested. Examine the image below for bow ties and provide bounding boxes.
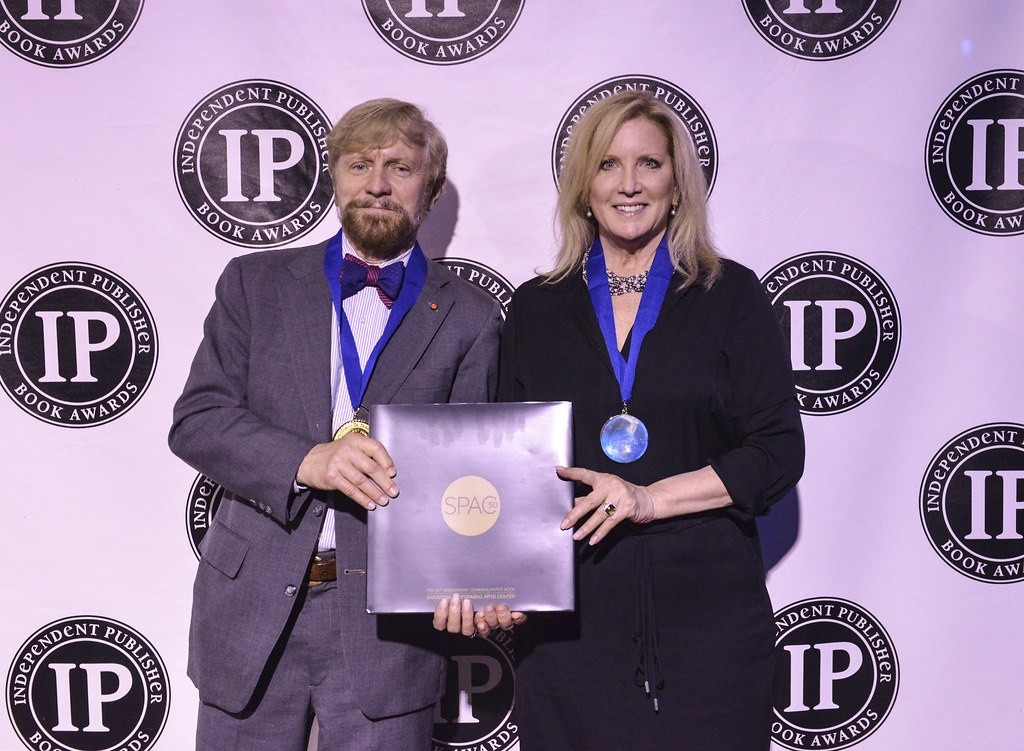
[342,253,403,308]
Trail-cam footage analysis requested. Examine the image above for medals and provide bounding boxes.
[327,419,370,445]
[599,412,649,464]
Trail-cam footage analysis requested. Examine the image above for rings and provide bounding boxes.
[489,624,500,630]
[469,627,477,639]
[500,624,514,630]
[602,503,617,517]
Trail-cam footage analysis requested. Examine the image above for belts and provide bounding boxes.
[303,556,336,586]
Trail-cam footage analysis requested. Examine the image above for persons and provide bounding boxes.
[168,97,473,750]
[468,88,805,750]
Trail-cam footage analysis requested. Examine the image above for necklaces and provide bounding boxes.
[581,240,649,296]
